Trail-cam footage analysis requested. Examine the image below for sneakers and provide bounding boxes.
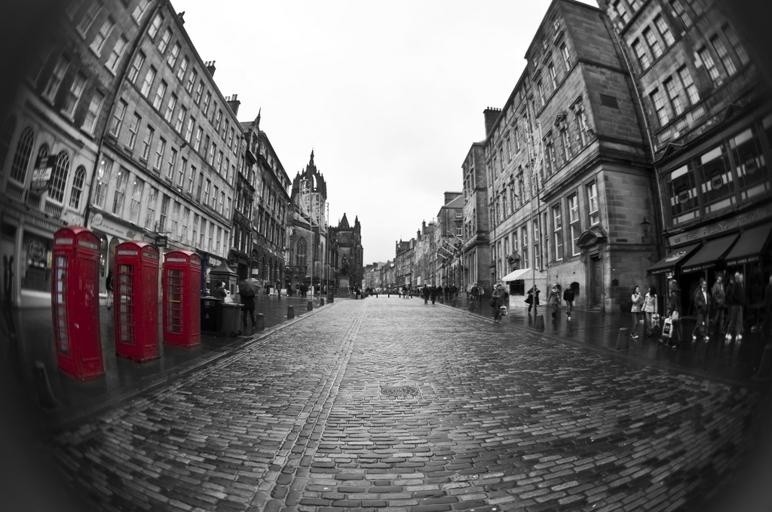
[692,333,743,340]
[630,334,639,339]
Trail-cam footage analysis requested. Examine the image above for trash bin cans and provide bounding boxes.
[219,303,244,337]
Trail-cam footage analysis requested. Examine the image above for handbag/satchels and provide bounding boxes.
[488,298,497,307]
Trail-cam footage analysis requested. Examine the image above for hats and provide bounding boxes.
[552,284,557,288]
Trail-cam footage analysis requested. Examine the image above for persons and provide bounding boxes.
[240,293,257,326]
[274,277,416,301]
[3,252,19,341]
[629,270,748,351]
[421,279,576,322]
[106,269,114,310]
[759,274,772,353]
[214,280,227,304]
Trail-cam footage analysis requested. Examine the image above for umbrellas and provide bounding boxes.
[239,277,263,298]
